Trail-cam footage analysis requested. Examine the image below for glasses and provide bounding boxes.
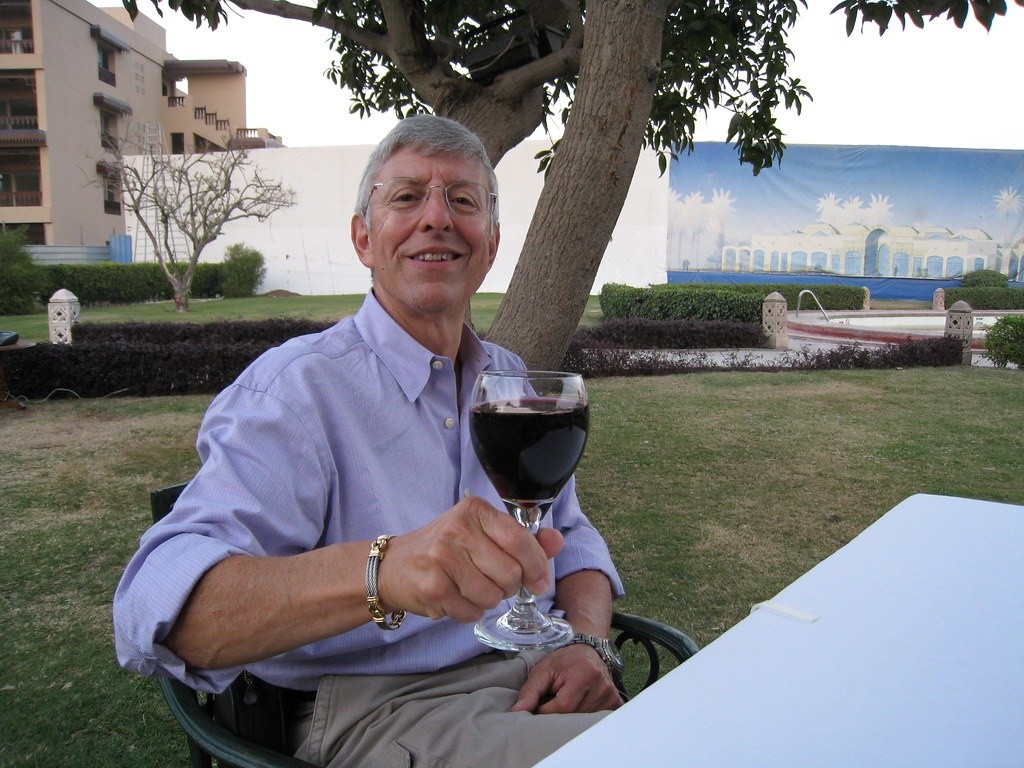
[362,177,498,218]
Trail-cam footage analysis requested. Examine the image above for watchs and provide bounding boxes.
[553,632,624,679]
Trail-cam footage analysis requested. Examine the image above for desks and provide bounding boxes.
[532,493,1024,768]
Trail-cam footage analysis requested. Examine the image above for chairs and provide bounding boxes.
[150,479,699,768]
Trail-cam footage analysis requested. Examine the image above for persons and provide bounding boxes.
[112,115,628,768]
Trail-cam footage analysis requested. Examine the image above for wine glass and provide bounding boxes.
[468,372,590,652]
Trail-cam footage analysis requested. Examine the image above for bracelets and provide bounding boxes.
[366,534,406,630]
